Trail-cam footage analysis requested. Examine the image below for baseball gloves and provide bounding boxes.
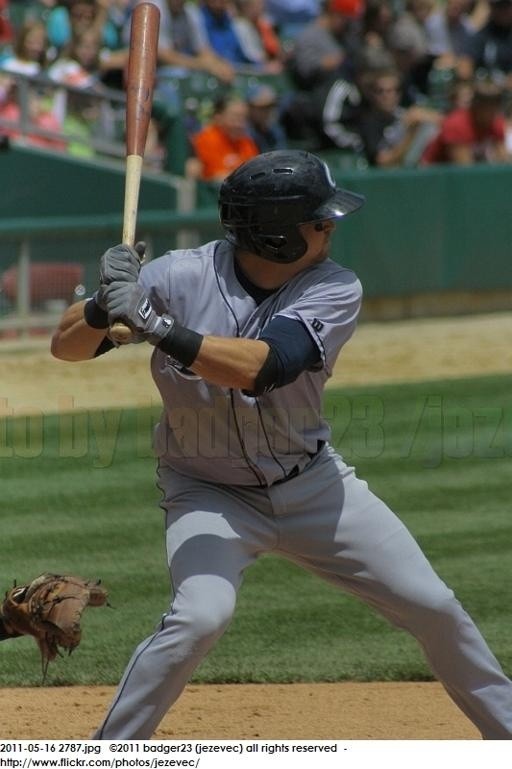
[0,572,108,663]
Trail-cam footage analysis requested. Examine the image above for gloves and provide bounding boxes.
[101,282,174,346]
[94,241,147,311]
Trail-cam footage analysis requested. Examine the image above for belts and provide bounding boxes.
[275,438,326,484]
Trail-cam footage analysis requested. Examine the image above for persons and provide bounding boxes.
[53,148,510,740]
[1,1,512,183]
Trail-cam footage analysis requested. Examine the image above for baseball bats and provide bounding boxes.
[109,4,162,344]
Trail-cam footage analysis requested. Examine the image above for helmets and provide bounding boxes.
[218,149,364,265]
